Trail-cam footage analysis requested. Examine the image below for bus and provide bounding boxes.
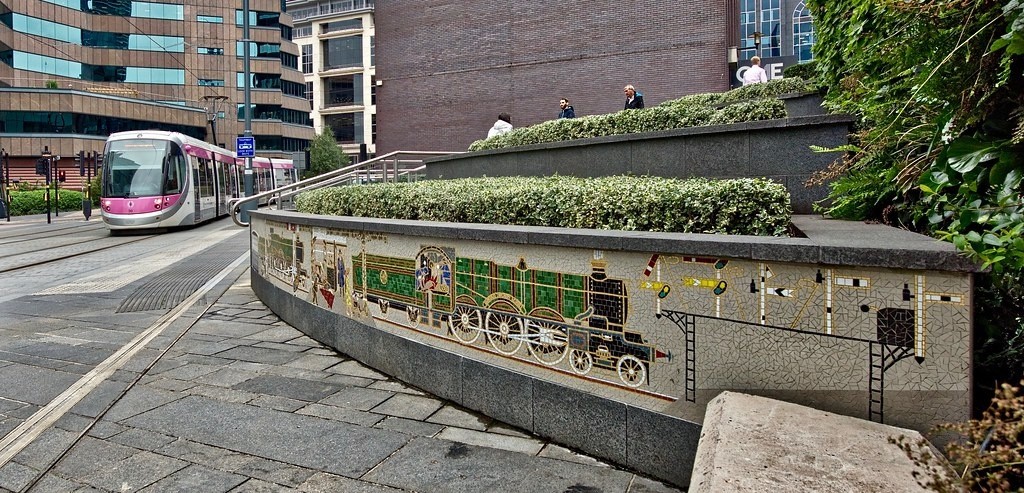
[100,96,294,229]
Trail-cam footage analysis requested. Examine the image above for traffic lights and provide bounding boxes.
[93,150,103,176]
[74,150,84,176]
[58,170,66,181]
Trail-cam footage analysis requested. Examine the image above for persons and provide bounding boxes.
[558,97,576,120]
[742,56,768,86]
[487,113,514,139]
[623,83,644,109]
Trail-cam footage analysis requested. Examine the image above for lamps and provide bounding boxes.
[377,81,382,86]
[736,32,764,50]
